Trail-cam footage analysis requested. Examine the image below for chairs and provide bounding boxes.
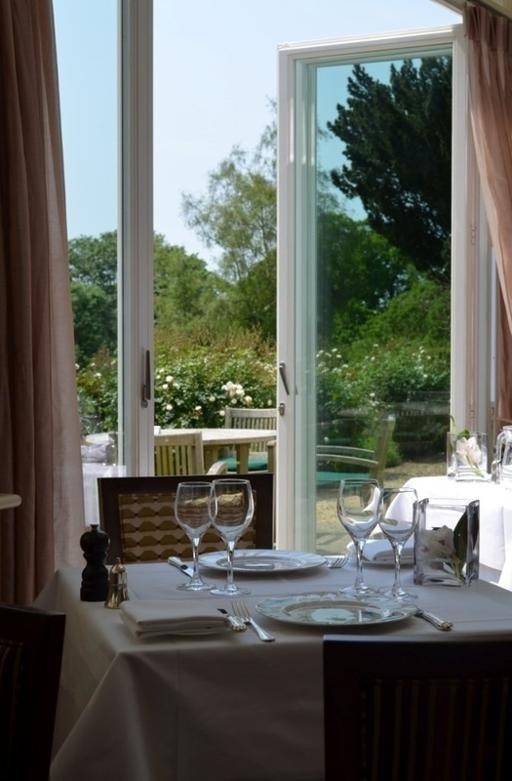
[321,635,508,775]
[155,432,228,476]
[98,473,278,560]
[315,415,396,509]
[0,604,67,768]
[218,406,277,473]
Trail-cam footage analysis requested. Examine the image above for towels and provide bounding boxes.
[347,538,417,567]
[119,595,230,642]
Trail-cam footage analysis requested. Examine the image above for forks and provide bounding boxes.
[324,554,350,569]
[228,598,276,642]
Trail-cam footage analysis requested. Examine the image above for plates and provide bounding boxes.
[256,590,419,626]
[199,548,327,573]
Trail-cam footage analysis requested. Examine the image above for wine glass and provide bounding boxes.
[336,479,383,593]
[211,479,254,595]
[378,488,421,600]
[174,481,217,592]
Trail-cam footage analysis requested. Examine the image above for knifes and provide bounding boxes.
[166,556,203,580]
[402,590,453,633]
